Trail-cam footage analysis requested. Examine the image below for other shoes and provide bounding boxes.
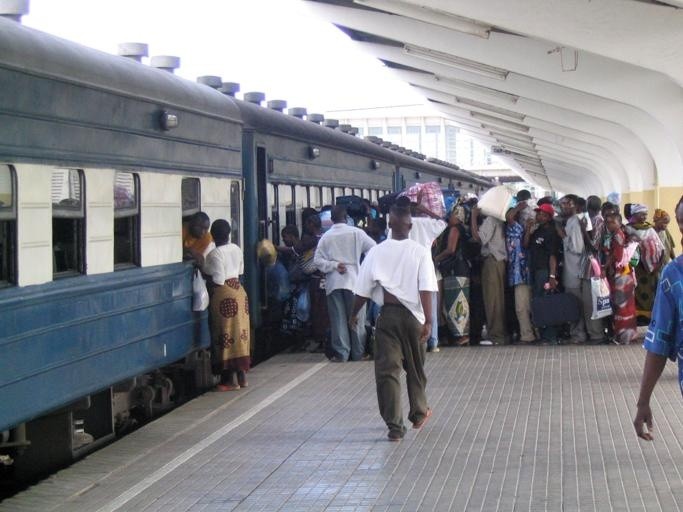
[512,338,536,345]
[586,336,608,345]
[331,356,348,361]
[454,335,469,345]
[537,338,560,346]
[430,346,440,353]
[360,354,373,360]
[479,336,504,345]
[559,338,584,345]
[609,333,638,345]
[286,345,326,353]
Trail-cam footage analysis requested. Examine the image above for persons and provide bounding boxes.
[182,210,215,266]
[259,182,674,363]
[346,199,440,442]
[195,222,251,391]
[631,193,683,441]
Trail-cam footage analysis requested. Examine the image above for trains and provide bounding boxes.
[0,0,501,470]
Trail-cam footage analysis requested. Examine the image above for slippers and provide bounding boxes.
[216,382,242,391]
[387,433,406,441]
[412,405,434,431]
[239,379,249,388]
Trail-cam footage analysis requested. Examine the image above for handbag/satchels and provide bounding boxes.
[588,275,614,322]
[529,286,582,328]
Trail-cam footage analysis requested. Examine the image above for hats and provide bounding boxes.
[534,203,555,217]
